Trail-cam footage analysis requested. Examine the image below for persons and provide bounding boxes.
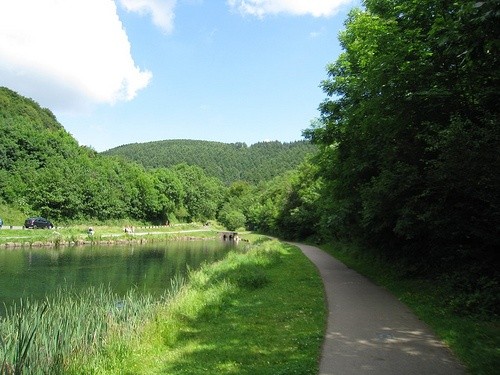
[130,226,135,234]
[87,226,94,236]
[124,226,129,233]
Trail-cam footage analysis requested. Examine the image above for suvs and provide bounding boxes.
[24,217,53,229]
